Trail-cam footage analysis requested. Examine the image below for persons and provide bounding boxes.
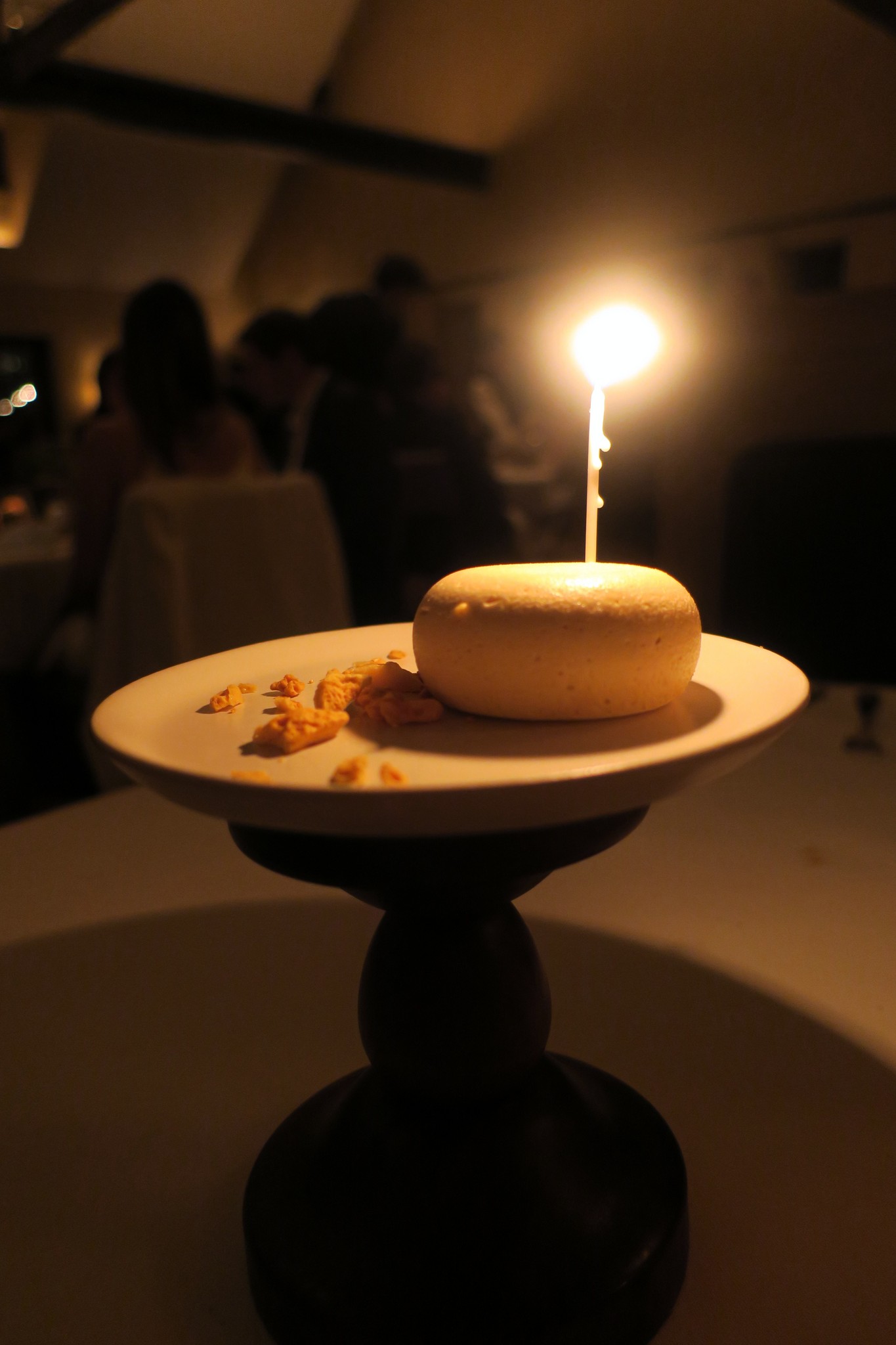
[75,253,515,626]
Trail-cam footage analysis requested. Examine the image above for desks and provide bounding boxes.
[2,680,896,1344]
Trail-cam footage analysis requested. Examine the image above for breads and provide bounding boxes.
[412,559,701,722]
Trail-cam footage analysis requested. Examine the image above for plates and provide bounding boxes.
[88,622,810,830]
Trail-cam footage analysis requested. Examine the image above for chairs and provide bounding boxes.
[61,476,347,785]
